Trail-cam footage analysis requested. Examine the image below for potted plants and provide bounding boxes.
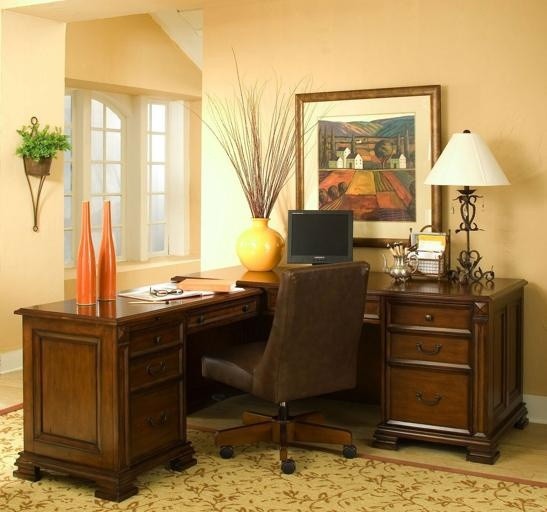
[14,120,74,178]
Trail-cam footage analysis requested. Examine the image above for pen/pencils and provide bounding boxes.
[127,301,170,304]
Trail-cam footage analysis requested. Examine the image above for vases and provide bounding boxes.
[235,217,285,272]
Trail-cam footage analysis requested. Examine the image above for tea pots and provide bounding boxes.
[382,253,418,283]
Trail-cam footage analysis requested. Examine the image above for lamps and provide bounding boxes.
[424,129,512,285]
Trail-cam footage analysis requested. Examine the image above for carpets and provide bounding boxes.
[0,400,546,512]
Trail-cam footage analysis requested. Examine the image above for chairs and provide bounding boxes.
[199,260,370,474]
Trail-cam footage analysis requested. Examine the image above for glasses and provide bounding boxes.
[149,287,183,297]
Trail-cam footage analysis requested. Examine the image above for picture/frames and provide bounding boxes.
[293,84,443,251]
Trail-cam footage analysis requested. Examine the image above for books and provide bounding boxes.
[116,288,246,303]
[175,278,236,294]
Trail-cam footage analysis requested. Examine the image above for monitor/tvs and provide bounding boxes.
[287,210,353,265]
